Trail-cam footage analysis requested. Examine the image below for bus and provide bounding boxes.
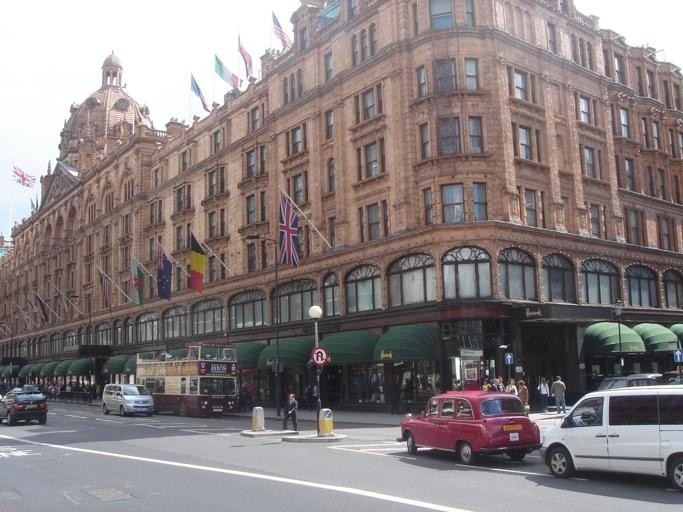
[135,342,241,416]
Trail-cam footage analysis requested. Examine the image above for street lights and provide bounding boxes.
[613,298,624,376]
[307,305,322,436]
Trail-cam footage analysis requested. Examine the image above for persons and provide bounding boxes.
[480,376,530,417]
[302,382,318,411]
[0,379,109,403]
[389,378,403,415]
[403,378,414,414]
[536,375,567,414]
[236,381,272,415]
[280,394,299,431]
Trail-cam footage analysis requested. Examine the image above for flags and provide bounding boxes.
[273,15,292,52]
[188,232,207,299]
[18,261,146,332]
[12,166,36,190]
[239,43,253,82]
[156,247,173,300]
[214,56,243,89]
[191,75,211,113]
[278,193,301,268]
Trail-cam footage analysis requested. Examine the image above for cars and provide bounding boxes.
[396,390,542,464]
[0,383,47,425]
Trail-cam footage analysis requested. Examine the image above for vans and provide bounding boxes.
[539,370,682,492]
[101,384,154,416]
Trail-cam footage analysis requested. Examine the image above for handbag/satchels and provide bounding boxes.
[523,405,530,410]
